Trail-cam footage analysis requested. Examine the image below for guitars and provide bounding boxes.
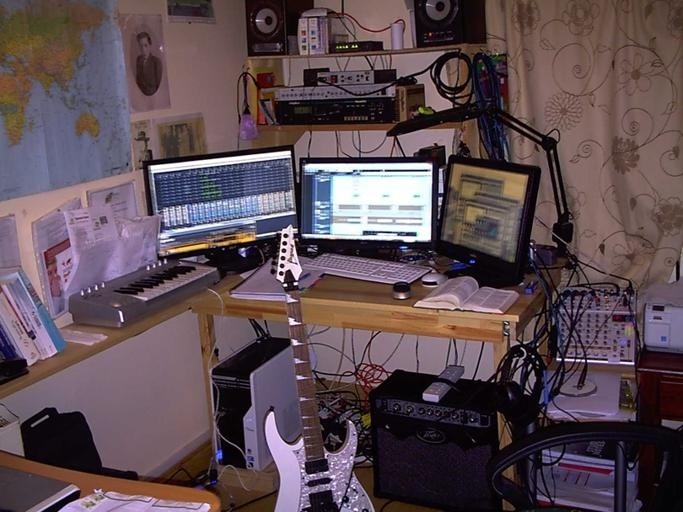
[263,223,375,512]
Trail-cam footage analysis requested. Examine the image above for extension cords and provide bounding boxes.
[422,365,465,403]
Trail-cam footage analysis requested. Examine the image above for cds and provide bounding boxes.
[559,379,598,397]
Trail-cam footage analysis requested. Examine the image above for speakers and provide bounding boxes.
[244,2,315,57]
[414,0,488,48]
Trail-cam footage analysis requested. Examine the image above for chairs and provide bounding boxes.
[486,420,683,512]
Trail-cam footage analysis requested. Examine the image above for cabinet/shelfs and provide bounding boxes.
[244,43,491,160]
[635,347,683,512]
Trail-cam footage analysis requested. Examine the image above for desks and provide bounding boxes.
[0,246,562,512]
[0,449,221,512]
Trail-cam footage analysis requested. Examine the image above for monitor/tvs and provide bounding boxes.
[437,155,542,288]
[298,157,438,249]
[142,144,301,276]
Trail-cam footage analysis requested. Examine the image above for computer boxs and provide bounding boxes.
[208,336,313,473]
[368,368,519,511]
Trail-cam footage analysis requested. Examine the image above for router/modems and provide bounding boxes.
[301,1,345,17]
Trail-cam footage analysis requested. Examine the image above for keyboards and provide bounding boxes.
[301,252,433,287]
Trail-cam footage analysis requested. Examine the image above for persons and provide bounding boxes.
[135,29,162,97]
[134,131,156,168]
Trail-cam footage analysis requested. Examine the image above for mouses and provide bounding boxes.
[421,273,446,287]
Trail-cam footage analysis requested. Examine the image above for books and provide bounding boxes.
[230,257,324,301]
[1,265,66,367]
[413,273,519,316]
